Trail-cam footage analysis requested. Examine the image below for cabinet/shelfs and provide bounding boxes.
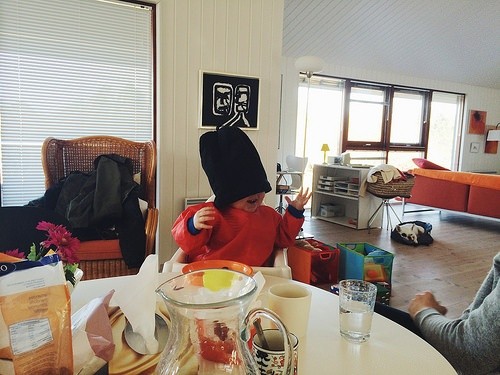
[311,164,384,230]
[333,181,359,198]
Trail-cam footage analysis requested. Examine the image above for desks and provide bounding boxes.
[0,274,458,375]
[276,170,303,195]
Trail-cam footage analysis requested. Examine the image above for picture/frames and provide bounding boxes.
[198,68,261,131]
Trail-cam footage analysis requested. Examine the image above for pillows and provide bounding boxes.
[412,158,451,172]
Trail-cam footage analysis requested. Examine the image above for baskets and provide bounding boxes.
[367,171,417,195]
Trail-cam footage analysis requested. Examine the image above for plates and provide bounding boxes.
[181,259,252,288]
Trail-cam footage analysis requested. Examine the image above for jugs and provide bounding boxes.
[155,268,262,375]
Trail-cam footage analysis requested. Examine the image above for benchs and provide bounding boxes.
[402,168,500,220]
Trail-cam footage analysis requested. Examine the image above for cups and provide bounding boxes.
[338,279,377,342]
[267,282,312,351]
[251,329,299,375]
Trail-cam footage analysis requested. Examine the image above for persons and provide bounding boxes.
[171,127,314,267]
[374,252,500,375]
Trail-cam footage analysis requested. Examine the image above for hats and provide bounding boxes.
[200,124,271,214]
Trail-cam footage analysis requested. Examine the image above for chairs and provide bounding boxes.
[162,193,292,281]
[275,155,307,215]
[41,136,159,282]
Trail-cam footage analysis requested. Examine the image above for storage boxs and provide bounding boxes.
[321,203,344,218]
[336,243,395,293]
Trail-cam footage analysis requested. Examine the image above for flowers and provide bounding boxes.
[0,220,83,288]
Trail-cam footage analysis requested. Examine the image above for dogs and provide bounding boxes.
[395,223,425,243]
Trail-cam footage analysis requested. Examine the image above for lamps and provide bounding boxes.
[295,55,324,156]
[487,122,500,142]
[321,144,329,165]
